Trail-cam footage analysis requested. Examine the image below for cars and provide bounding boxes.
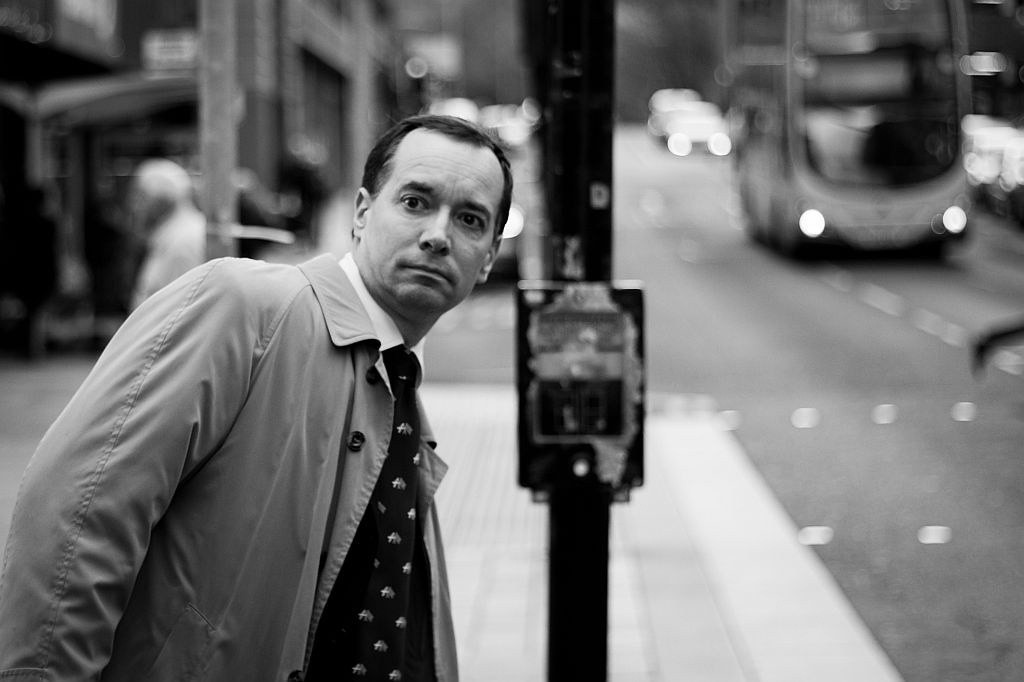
[651,89,724,152]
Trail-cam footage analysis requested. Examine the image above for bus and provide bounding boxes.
[725,1,971,258]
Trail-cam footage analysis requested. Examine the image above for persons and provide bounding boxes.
[0,114,514,682]
[1,130,331,367]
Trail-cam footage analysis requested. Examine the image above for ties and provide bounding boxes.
[349,349,421,682]
